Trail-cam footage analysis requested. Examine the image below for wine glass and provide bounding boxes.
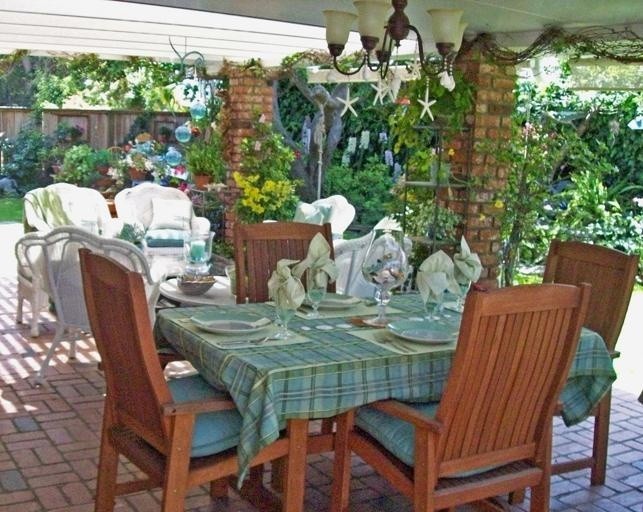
[272,278,296,340]
[448,262,471,322]
[182,230,215,276]
[420,278,443,327]
[305,268,328,319]
[361,228,410,329]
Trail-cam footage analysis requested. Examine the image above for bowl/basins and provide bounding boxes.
[177,276,218,296]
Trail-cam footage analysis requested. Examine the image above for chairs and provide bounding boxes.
[543,239,638,487]
[79,248,288,510]
[14,180,237,361]
[333,282,591,511]
[234,222,338,490]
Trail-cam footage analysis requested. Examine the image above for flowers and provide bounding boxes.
[106,151,155,180]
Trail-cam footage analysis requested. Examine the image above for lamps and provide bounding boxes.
[322,0,468,93]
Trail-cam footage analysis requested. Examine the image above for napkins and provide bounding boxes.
[268,259,306,311]
[291,231,339,289]
[453,235,483,285]
[416,250,462,304]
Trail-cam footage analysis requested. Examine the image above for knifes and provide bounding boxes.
[217,338,279,345]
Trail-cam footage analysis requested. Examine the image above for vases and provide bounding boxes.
[127,166,148,181]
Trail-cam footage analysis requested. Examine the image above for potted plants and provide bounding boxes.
[180,137,226,191]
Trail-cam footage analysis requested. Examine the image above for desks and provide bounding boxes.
[154,296,601,509]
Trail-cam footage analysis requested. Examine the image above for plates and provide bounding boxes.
[301,293,361,311]
[387,319,458,346]
[190,311,271,336]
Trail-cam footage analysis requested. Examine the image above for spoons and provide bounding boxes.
[221,336,270,346]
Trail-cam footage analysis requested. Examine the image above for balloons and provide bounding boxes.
[175,125,191,143]
[165,150,183,166]
[190,101,206,119]
